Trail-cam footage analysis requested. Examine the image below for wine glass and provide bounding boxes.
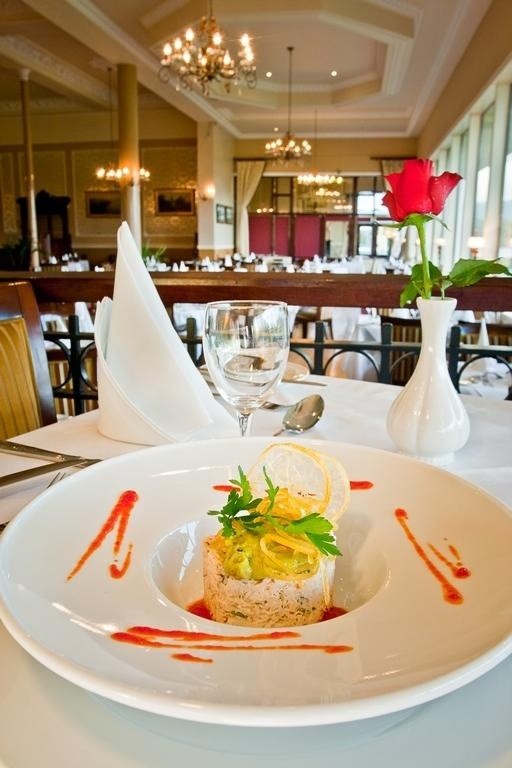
[203,299,288,437]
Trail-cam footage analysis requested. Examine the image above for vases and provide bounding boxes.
[387,296,471,459]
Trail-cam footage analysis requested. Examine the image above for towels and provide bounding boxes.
[173,260,189,273]
[313,254,323,274]
[49,256,56,265]
[201,255,209,268]
[94,221,244,443]
[477,317,490,350]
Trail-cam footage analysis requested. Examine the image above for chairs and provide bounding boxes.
[48,346,97,418]
[0,281,58,443]
[458,319,512,362]
[380,316,423,385]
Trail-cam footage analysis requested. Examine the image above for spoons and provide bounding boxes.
[269,394,324,438]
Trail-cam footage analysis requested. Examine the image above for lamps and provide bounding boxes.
[312,187,349,208]
[265,46,312,166]
[297,107,343,188]
[94,67,151,187]
[157,1,259,96]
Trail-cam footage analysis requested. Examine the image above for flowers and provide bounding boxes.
[381,156,512,308]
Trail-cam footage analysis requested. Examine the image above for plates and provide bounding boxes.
[0,437,512,727]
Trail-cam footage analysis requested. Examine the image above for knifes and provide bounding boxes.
[0,457,82,487]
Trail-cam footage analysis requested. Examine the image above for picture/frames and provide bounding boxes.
[154,188,196,216]
[85,190,122,219]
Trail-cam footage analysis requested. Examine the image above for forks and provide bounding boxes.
[0,472,72,534]
